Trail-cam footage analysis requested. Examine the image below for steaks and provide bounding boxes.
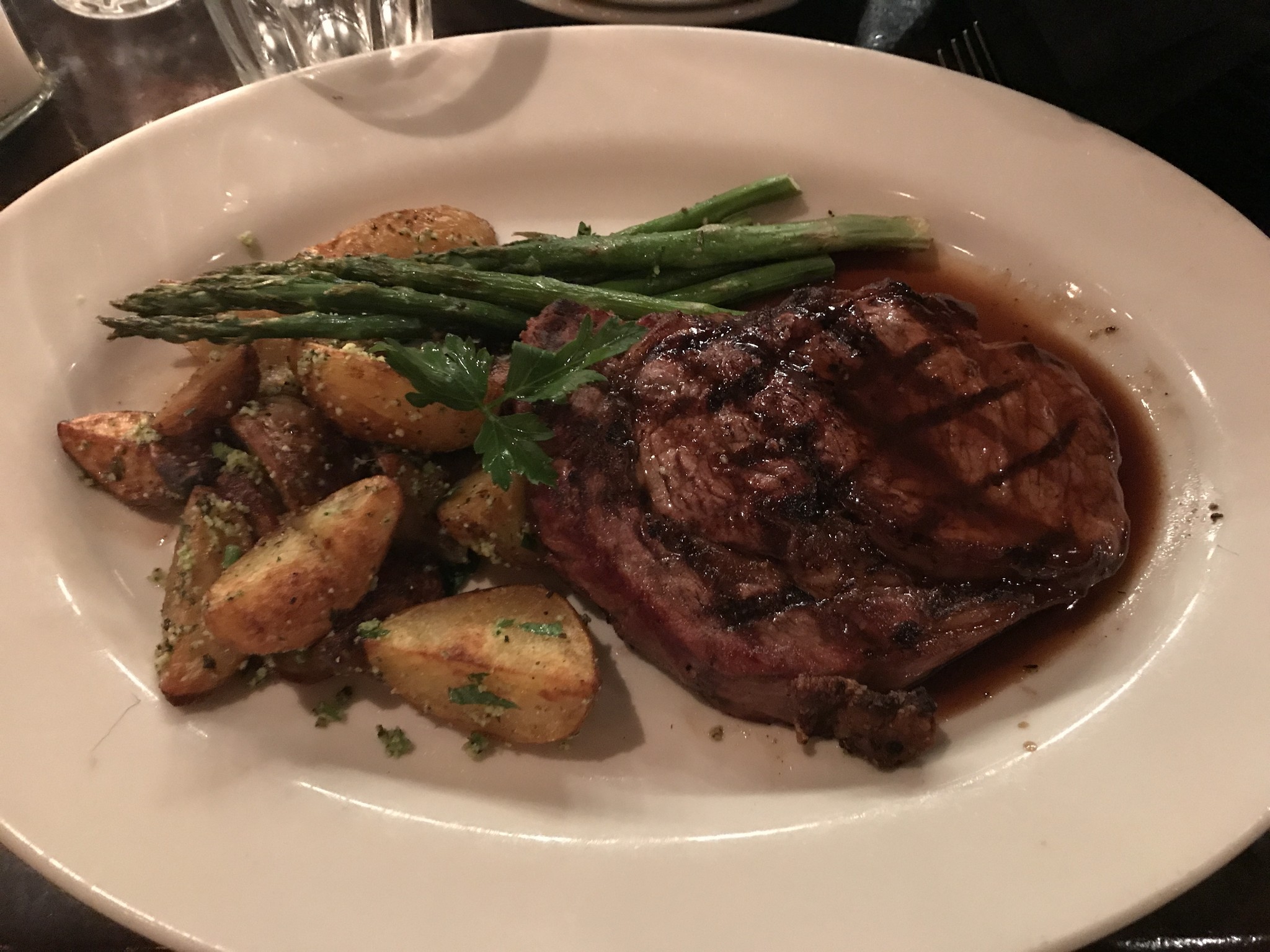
[502,295,1130,777]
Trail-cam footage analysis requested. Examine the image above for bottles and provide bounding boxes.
[0,3,58,139]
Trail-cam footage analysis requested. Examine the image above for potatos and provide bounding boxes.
[57,202,599,749]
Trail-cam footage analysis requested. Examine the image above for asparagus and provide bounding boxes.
[98,181,938,341]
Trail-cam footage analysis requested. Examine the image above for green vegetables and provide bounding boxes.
[365,320,648,483]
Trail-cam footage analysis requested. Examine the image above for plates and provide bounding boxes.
[520,0,804,26]
[0,14,1270,952]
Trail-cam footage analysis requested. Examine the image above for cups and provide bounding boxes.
[52,0,179,19]
[204,0,433,88]
